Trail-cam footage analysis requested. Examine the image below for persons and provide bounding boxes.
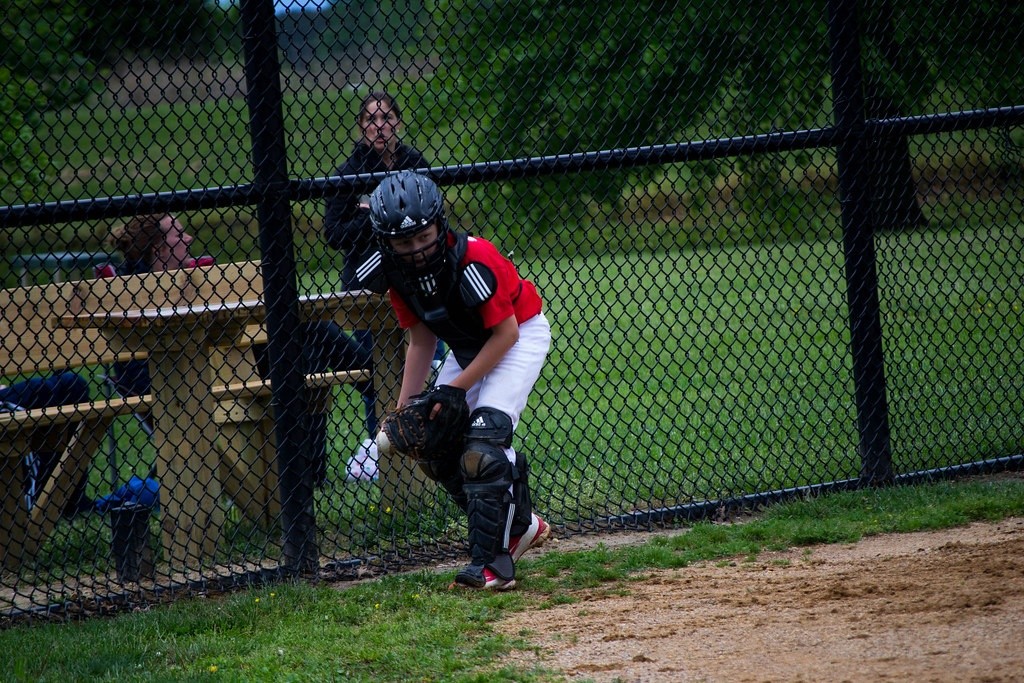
[0,373,96,520]
[367,172,551,589]
[111,213,374,490]
[323,92,445,371]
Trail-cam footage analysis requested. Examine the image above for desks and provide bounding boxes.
[50,288,428,579]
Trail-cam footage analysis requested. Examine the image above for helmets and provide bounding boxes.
[369,171,450,297]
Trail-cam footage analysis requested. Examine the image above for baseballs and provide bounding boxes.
[376,430,391,452]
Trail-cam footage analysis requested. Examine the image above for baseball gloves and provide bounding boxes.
[382,384,469,463]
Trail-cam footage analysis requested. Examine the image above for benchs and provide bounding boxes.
[0,257,372,582]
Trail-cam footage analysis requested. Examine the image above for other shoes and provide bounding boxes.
[449,566,517,591]
[506,512,551,564]
[66,492,93,517]
